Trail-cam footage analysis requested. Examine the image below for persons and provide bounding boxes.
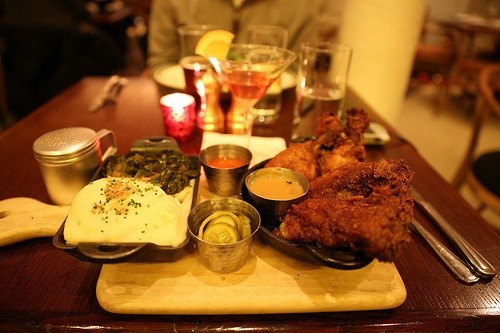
[146,0,348,75]
[85,0,148,59]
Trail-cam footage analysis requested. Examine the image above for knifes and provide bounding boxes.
[87,75,120,112]
[409,185,497,280]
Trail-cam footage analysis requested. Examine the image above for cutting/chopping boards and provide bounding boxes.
[0,161,407,315]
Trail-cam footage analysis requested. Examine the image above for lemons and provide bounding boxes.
[194,29,235,61]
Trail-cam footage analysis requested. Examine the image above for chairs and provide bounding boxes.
[410,0,500,217]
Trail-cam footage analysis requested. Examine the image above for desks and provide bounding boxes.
[439,20,500,57]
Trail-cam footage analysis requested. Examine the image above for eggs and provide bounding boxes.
[63,175,188,247]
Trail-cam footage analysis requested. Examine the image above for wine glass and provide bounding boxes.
[208,43,296,136]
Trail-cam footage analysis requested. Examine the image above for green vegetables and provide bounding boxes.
[92,149,200,233]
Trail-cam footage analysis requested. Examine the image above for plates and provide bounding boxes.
[53,148,196,260]
[151,63,296,93]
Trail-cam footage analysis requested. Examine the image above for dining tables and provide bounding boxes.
[0,76,500,333]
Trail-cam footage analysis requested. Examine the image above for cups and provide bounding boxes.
[32,126,118,204]
[177,24,219,114]
[244,167,310,226]
[291,40,353,142]
[245,23,288,125]
[188,197,261,274]
[199,144,252,197]
[191,61,224,131]
[159,93,196,144]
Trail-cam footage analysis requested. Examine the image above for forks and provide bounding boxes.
[102,78,127,106]
